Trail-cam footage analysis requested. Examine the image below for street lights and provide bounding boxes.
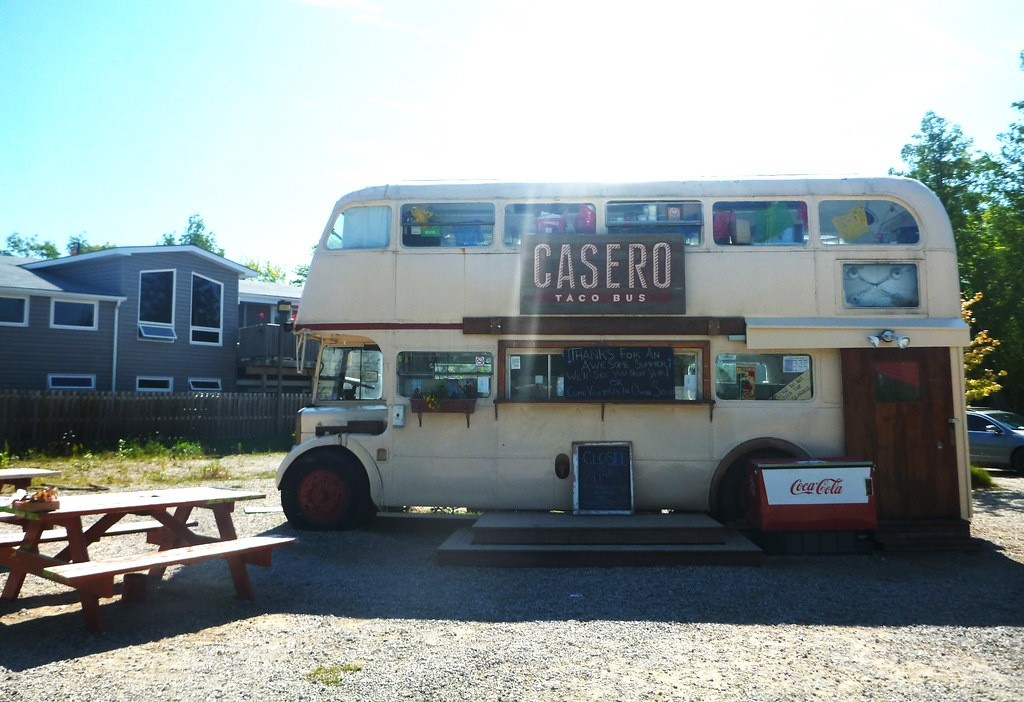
[274,298,292,449]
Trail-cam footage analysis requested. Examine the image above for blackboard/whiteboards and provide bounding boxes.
[571,440,634,516]
[563,344,676,403]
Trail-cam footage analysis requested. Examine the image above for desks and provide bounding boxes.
[0,468,61,490]
[0,485,255,635]
[744,461,879,557]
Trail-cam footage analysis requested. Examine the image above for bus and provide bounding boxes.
[273,176,976,555]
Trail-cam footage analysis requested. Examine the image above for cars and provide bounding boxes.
[965,405,1024,476]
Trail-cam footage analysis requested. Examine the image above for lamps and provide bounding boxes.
[869,331,909,349]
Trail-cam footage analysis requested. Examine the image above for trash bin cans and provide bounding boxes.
[747,459,876,532]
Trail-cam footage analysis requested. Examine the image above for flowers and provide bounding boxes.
[407,379,475,412]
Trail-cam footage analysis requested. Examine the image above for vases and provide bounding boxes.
[411,398,477,414]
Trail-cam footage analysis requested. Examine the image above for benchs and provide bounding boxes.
[0,519,296,634]
